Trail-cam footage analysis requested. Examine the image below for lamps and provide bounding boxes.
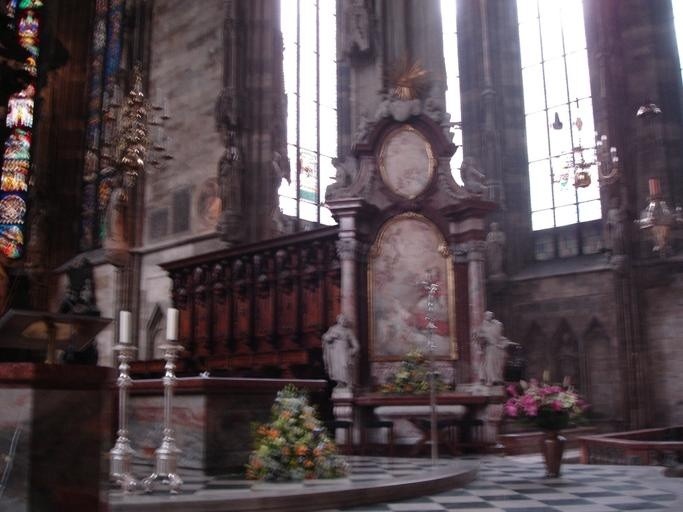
[101,57,173,188]
[550,119,619,188]
[636,103,662,119]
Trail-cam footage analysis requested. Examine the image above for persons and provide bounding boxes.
[473,311,520,384]
[103,176,129,247]
[486,221,507,279]
[216,131,245,241]
[606,196,630,259]
[322,316,362,385]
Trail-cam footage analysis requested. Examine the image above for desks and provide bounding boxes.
[352,394,488,457]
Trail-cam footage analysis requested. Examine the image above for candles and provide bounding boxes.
[120,310,133,344]
[649,179,662,195]
[166,308,179,341]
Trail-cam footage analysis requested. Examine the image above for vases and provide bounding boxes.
[539,429,566,476]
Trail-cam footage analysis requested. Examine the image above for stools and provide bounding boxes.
[374,404,465,458]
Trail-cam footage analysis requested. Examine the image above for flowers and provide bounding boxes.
[246,383,353,481]
[382,348,451,394]
[504,370,589,429]
